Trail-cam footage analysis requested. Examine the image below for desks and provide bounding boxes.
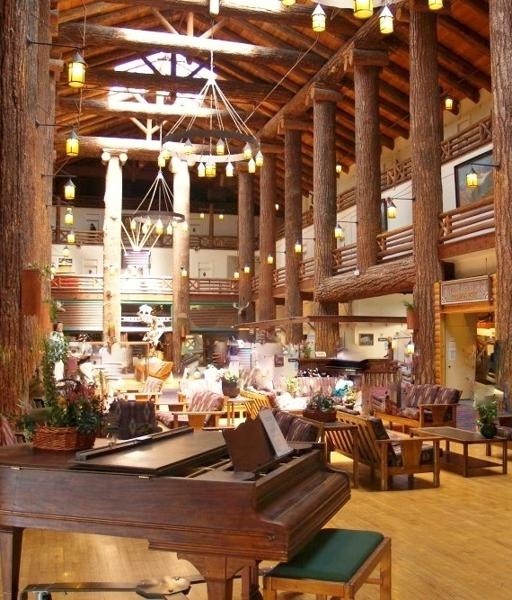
[289,408,359,490]
[404,422,511,478]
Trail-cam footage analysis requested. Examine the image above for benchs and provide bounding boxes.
[325,403,446,492]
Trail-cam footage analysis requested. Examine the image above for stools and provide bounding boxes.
[260,525,394,599]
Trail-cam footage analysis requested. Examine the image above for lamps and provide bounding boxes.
[294,237,307,254]
[439,88,455,111]
[352,0,374,20]
[385,196,398,220]
[64,207,74,228]
[67,230,76,245]
[378,4,396,36]
[178,266,190,279]
[98,146,131,169]
[310,3,327,33]
[151,75,265,179]
[200,210,205,220]
[334,160,342,174]
[218,212,224,223]
[242,261,257,275]
[267,249,281,265]
[428,0,443,11]
[65,52,86,90]
[232,268,244,281]
[63,131,79,157]
[122,173,187,237]
[334,219,348,239]
[60,245,71,256]
[466,160,482,191]
[62,178,77,202]
[279,0,297,8]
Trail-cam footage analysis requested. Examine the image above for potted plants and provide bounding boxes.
[20,331,103,452]
[468,396,501,439]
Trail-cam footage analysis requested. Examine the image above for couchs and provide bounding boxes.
[250,401,323,445]
[370,383,463,425]
[156,387,230,430]
